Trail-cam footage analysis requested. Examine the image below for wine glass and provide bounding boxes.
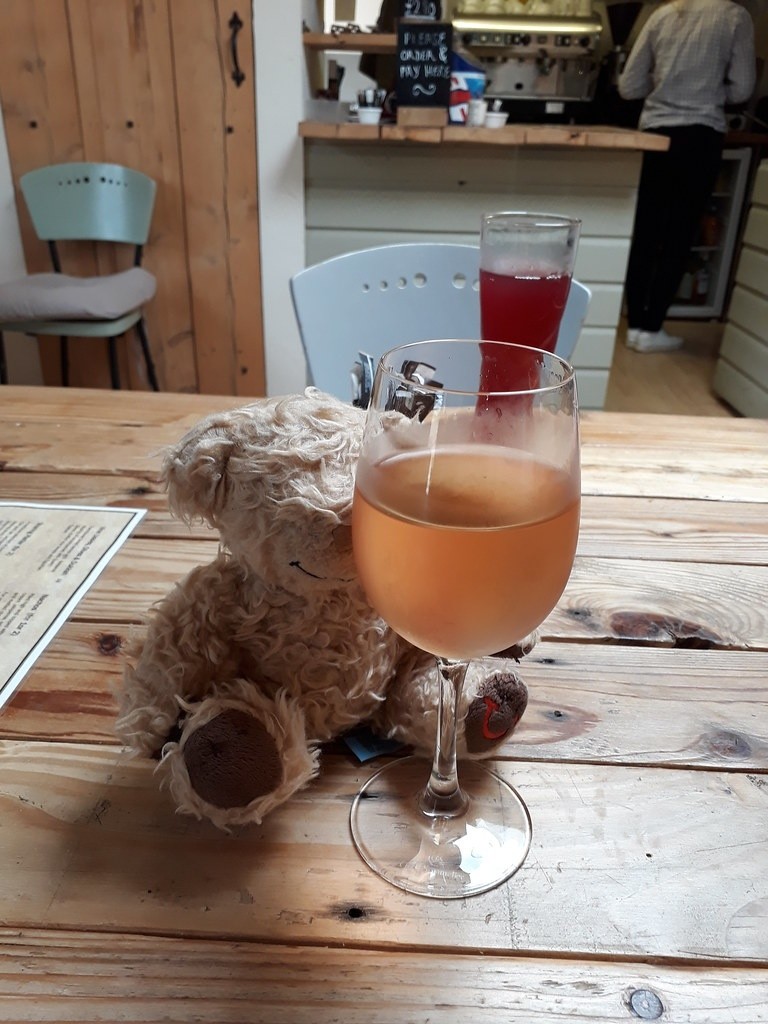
[349,338,581,900]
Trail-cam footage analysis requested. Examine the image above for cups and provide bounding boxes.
[357,107,383,124]
[465,99,488,127]
[475,211,582,418]
[485,112,509,128]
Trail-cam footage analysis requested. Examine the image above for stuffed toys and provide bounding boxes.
[114,390,539,830]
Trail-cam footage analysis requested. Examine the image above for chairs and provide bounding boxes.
[0,162,160,392]
[289,241,600,411]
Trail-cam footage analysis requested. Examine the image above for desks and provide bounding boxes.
[0,385,768,1024]
[301,123,671,413]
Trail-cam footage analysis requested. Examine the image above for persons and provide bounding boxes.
[620,0,755,352]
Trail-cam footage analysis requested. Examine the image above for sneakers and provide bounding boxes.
[626,328,683,353]
